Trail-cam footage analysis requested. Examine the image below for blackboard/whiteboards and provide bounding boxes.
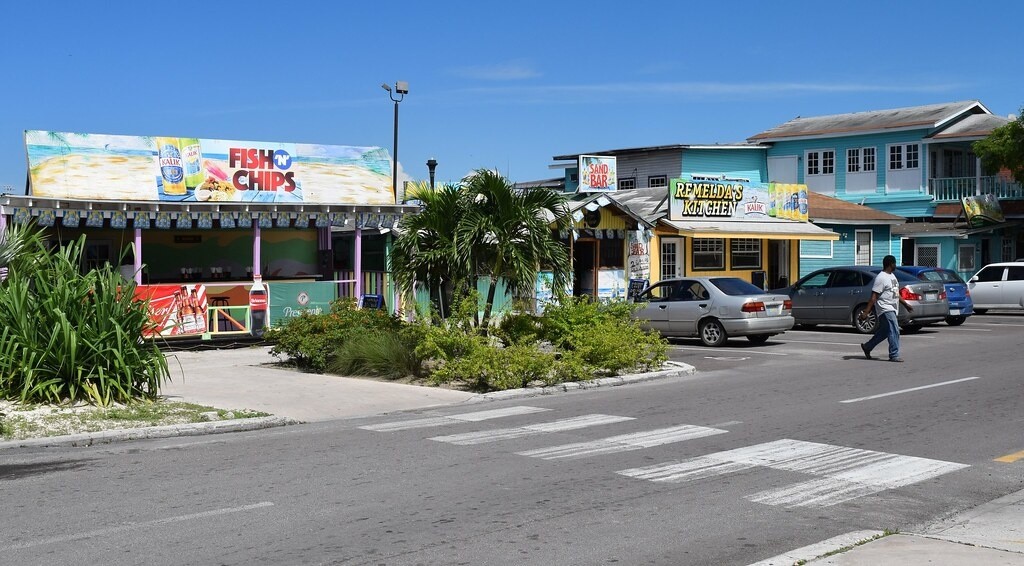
[626,278,651,301]
[356,294,389,315]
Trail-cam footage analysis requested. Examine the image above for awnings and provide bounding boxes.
[654,214,841,243]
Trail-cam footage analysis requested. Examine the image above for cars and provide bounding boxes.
[623,276,796,348]
[966,261,1024,314]
[762,265,949,335]
[895,266,973,327]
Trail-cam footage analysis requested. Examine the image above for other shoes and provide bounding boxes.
[860,343,873,359]
[889,356,904,362]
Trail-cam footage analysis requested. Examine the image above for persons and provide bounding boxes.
[858,253,917,363]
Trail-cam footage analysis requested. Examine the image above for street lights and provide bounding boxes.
[427,157,438,195]
